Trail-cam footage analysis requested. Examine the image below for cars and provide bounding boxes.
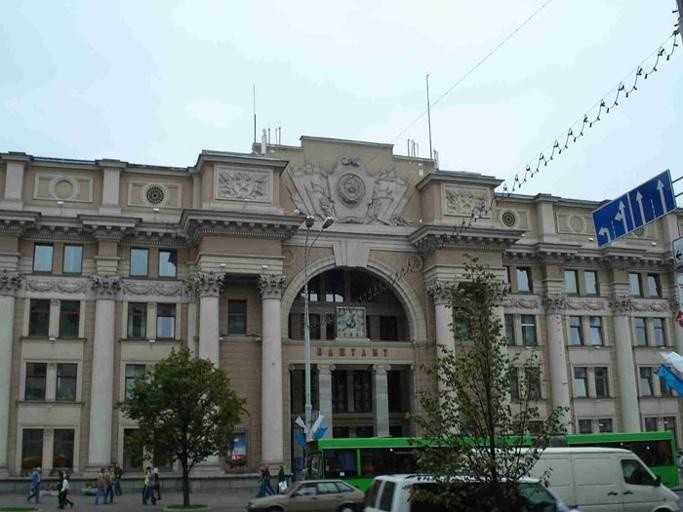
[245,479,366,512]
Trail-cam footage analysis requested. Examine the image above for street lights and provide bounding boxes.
[303,215,334,478]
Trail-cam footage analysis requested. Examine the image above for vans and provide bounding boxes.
[363,474,581,512]
[452,446,683,512]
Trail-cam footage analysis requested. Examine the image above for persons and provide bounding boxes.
[115,464,123,496]
[142,466,162,505]
[57,471,74,509]
[94,468,115,505]
[277,465,285,495]
[27,468,42,503]
[255,464,275,497]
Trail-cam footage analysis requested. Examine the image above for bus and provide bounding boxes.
[304,431,681,494]
